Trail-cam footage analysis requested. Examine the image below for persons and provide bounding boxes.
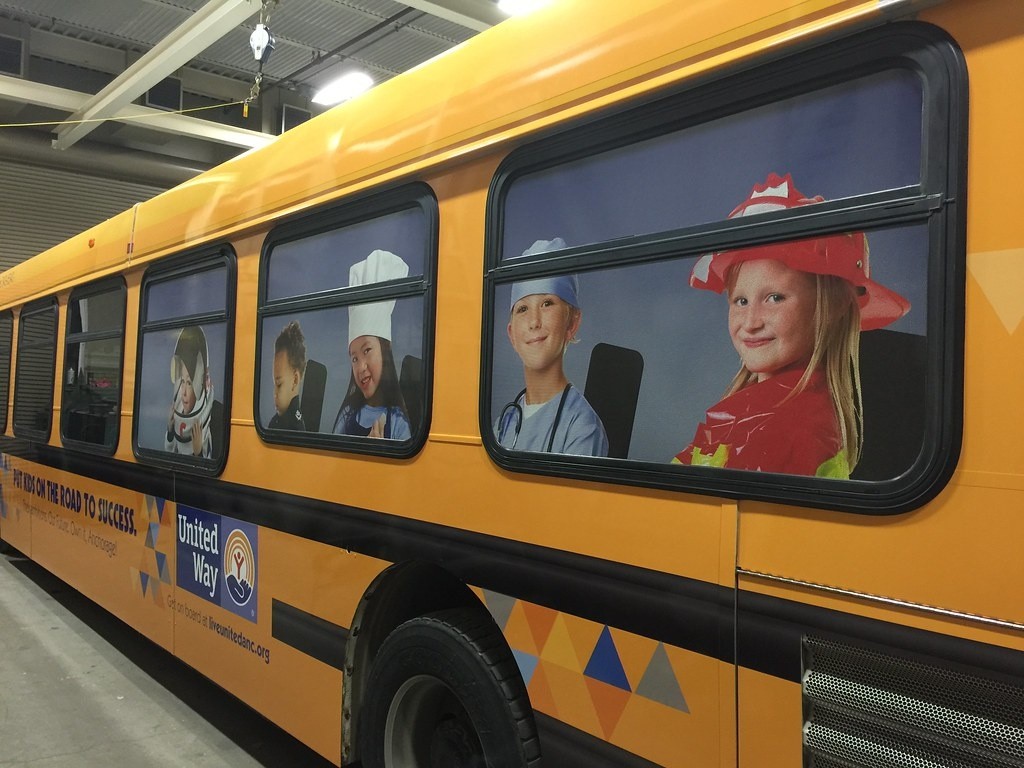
[267,321,306,432]
[493,236,610,456]
[164,324,214,457]
[664,175,908,484]
[333,250,414,441]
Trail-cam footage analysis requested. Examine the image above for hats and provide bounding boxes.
[508,238,579,310]
[347,249,410,348]
[688,172,911,331]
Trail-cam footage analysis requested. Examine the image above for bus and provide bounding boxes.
[0,2,1024,768]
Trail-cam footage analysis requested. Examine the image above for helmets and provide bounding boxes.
[171,325,214,443]
